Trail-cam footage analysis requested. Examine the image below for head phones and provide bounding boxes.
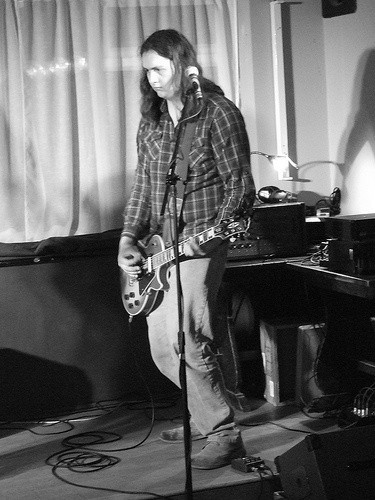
[258,185,298,203]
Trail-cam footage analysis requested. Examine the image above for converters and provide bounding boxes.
[233,457,263,472]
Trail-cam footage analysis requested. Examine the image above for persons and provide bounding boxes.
[117,29,256,468]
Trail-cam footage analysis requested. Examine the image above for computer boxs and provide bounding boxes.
[258,320,331,408]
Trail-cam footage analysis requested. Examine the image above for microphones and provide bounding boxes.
[187,65,205,99]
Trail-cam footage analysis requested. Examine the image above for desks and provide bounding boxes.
[216,252,375,412]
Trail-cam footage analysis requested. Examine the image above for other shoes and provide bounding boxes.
[190,439,248,470]
[158,423,205,443]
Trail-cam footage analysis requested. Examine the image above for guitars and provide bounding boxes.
[120,214,252,316]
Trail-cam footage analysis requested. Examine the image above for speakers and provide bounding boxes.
[273,425,375,500]
